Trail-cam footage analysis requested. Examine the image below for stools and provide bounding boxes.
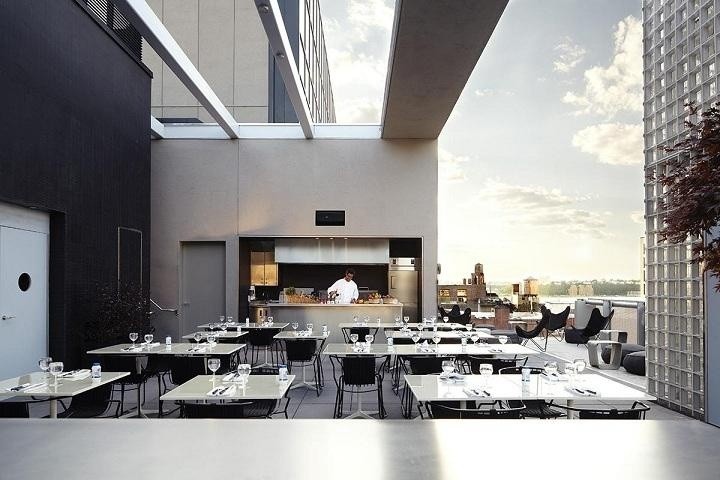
[600,329,628,350]
[586,340,623,369]
[621,351,647,376]
[602,344,645,362]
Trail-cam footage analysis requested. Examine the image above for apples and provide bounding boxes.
[367,293,381,299]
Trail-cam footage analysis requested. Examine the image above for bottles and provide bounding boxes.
[92,363,101,379]
[279,364,288,381]
[320,298,335,304]
[521,366,531,384]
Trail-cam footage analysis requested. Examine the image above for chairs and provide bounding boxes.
[497,356,528,369]
[41,358,122,418]
[469,354,517,410]
[175,401,254,418]
[513,310,551,351]
[161,356,212,416]
[143,305,475,399]
[541,307,570,341]
[97,356,146,419]
[408,356,462,407]
[329,354,390,420]
[424,401,527,420]
[2,401,31,418]
[579,401,650,419]
[222,367,289,419]
[564,308,615,349]
[498,367,562,420]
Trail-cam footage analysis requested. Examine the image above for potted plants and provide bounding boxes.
[285,286,297,303]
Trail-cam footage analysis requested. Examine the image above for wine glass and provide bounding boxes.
[545,358,587,387]
[480,364,494,389]
[38,356,65,386]
[207,359,251,389]
[441,360,454,384]
[128,316,329,352]
[350,316,508,351]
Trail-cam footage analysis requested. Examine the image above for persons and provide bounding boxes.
[326,268,360,304]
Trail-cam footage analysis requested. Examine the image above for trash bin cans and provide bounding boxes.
[249,304,271,322]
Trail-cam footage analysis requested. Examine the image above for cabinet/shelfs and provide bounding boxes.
[250,251,278,287]
